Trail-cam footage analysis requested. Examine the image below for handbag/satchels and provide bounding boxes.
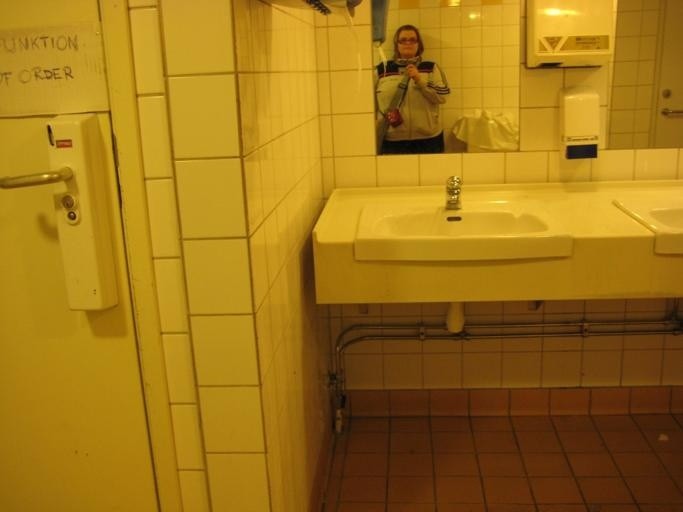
[376,122,388,155]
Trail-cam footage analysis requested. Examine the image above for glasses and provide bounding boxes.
[398,40,418,44]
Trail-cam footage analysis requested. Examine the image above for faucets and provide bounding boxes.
[446,175,463,210]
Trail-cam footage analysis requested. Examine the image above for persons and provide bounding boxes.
[373,23,452,154]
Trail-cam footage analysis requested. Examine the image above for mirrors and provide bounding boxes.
[373,1,521,150]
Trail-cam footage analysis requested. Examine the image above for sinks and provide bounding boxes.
[613,196,682,255]
[354,203,573,262]
